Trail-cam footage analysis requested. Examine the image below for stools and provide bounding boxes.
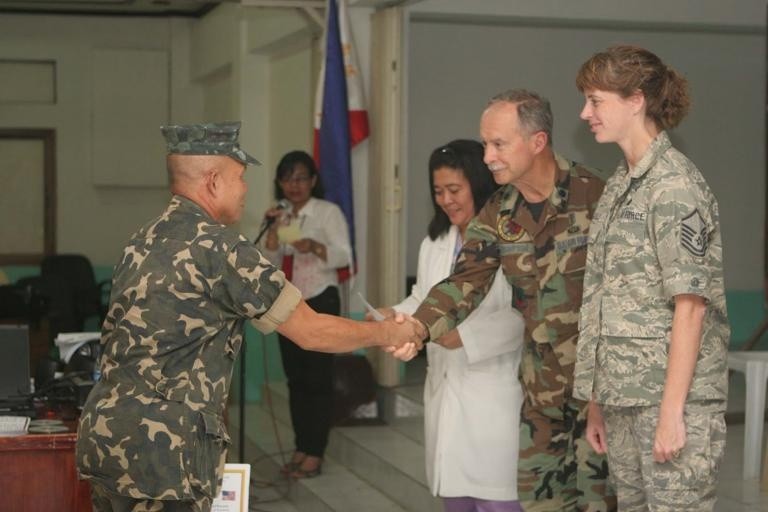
[726,342,767,487]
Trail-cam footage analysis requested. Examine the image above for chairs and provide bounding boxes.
[36,248,116,328]
[1,274,87,382]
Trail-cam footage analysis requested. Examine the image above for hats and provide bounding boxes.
[160,120,261,167]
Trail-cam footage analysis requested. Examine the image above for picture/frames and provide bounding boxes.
[0,122,60,272]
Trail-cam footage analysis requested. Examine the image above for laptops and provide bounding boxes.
[0,324,35,416]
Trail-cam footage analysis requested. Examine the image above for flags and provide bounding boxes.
[312,1,371,283]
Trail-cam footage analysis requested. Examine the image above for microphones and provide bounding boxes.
[267,196,292,226]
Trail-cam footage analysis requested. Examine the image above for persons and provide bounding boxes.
[73,121,429,512]
[364,139,527,510]
[570,45,732,511]
[262,149,353,479]
[378,88,619,512]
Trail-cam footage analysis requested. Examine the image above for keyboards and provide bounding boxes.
[0,415,32,435]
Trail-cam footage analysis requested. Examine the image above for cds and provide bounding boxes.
[29,419,64,425]
[29,425,70,434]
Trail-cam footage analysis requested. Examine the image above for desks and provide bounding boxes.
[0,395,97,512]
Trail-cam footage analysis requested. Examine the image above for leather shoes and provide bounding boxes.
[281,462,321,478]
